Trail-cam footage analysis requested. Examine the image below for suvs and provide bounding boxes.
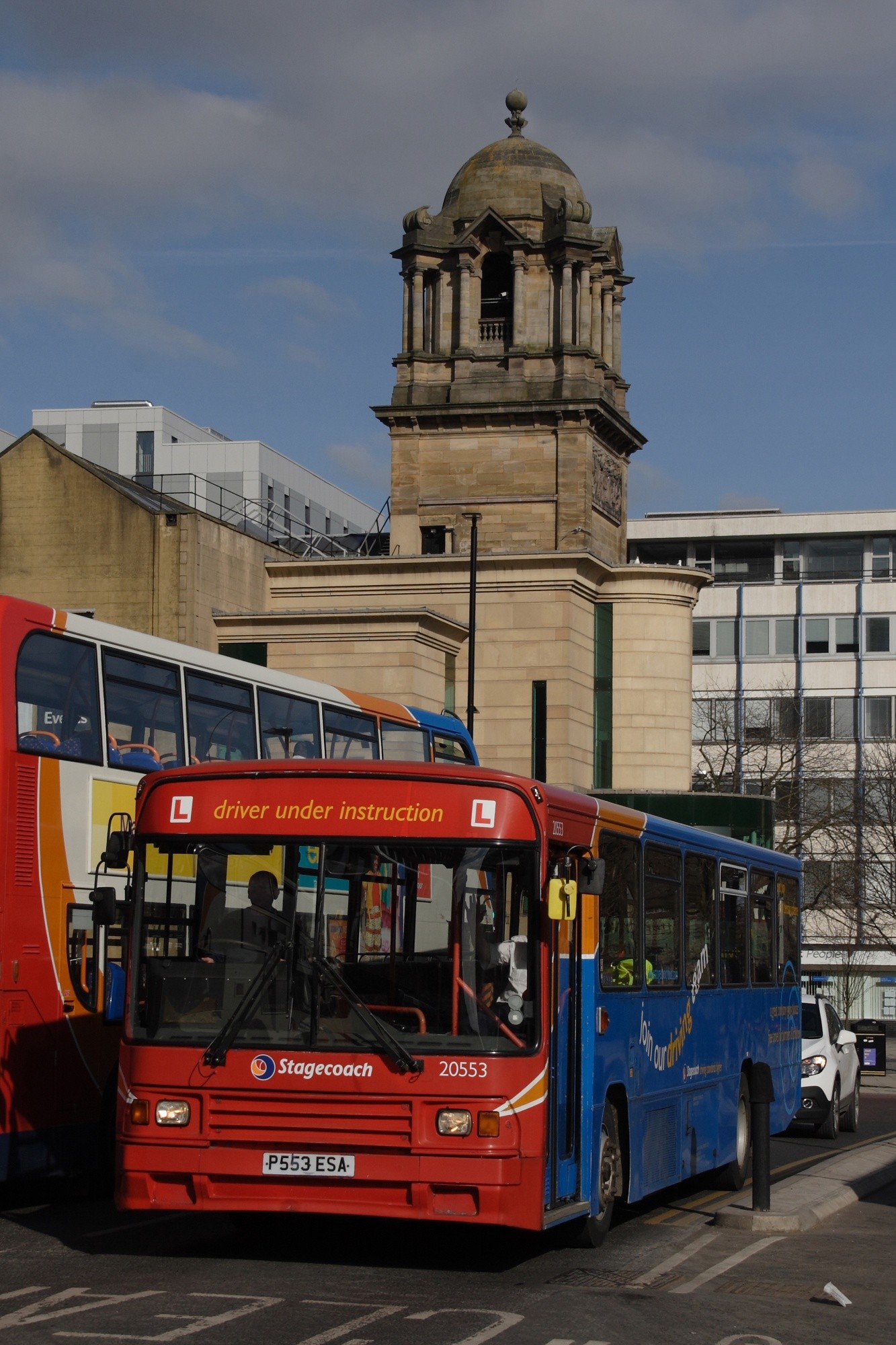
[780,994,861,1140]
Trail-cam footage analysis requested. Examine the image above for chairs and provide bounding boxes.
[19,729,201,772]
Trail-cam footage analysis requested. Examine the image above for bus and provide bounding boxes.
[0,593,479,1171]
[87,758,806,1247]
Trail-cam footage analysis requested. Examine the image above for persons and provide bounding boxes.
[197,870,300,963]
[291,740,314,759]
[463,892,528,1019]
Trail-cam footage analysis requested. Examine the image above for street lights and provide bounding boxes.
[464,511,483,741]
